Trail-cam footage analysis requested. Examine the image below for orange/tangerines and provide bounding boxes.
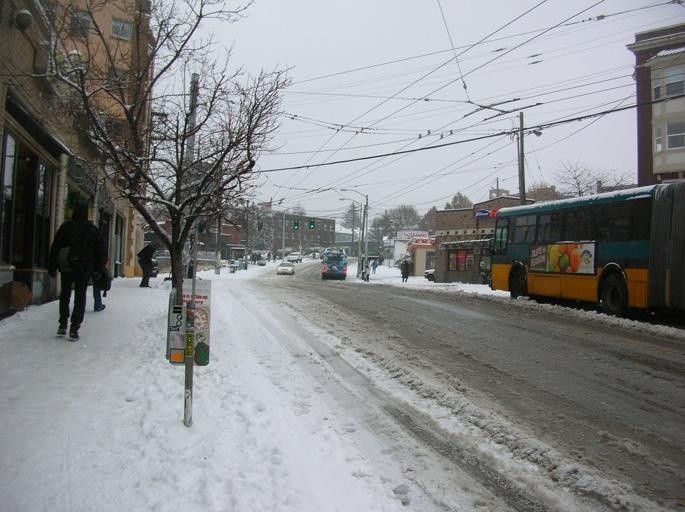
[559,254,569,268]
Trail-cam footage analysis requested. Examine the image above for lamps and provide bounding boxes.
[9,9,32,30]
[68,49,83,64]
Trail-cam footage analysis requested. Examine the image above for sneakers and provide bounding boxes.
[56,328,66,336]
[96,304,105,311]
[69,331,79,341]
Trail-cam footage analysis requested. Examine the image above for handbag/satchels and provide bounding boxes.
[100,267,111,290]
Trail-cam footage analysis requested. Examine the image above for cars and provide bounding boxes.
[287,252,303,263]
[277,262,295,275]
[423,268,434,281]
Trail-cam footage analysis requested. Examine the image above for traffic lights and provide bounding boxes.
[294,220,299,230]
[309,218,315,230]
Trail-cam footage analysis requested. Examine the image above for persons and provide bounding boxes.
[47,200,106,339]
[137,240,160,288]
[401,260,409,283]
[92,254,109,312]
[250,251,276,265]
[362,258,378,282]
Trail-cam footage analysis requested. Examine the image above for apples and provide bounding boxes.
[570,249,581,271]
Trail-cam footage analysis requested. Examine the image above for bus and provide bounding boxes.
[489,179,684,319]
[321,251,348,280]
